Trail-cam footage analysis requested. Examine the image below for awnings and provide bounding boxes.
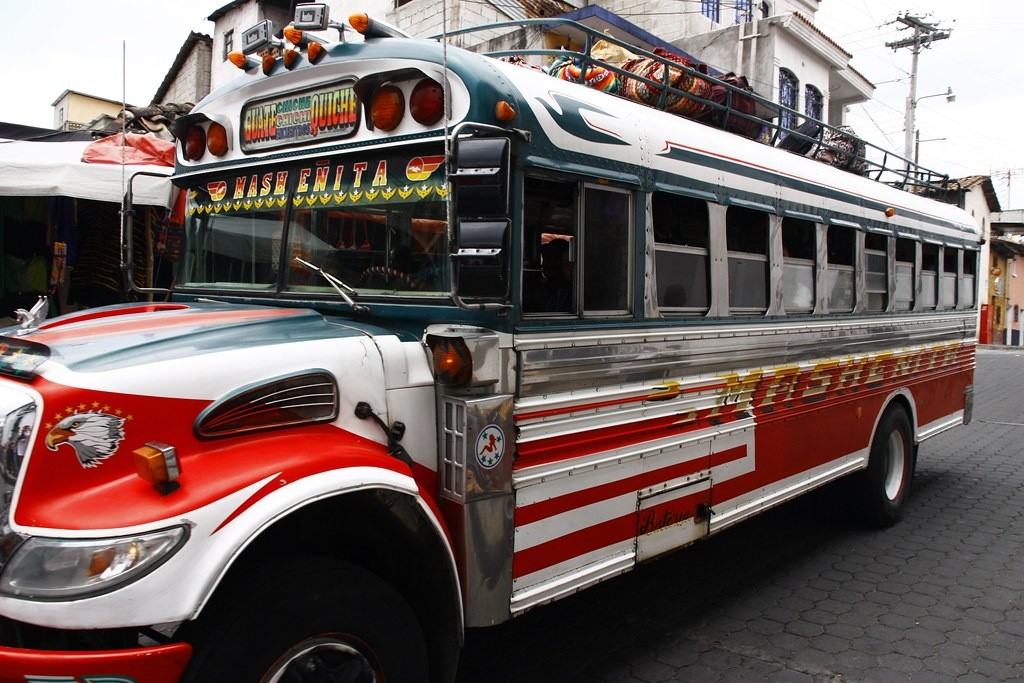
[990,233,1024,251]
[0,138,179,210]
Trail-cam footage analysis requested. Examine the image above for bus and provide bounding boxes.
[0,2,994,682]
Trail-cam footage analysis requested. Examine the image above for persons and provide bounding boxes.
[991,267,1003,325]
